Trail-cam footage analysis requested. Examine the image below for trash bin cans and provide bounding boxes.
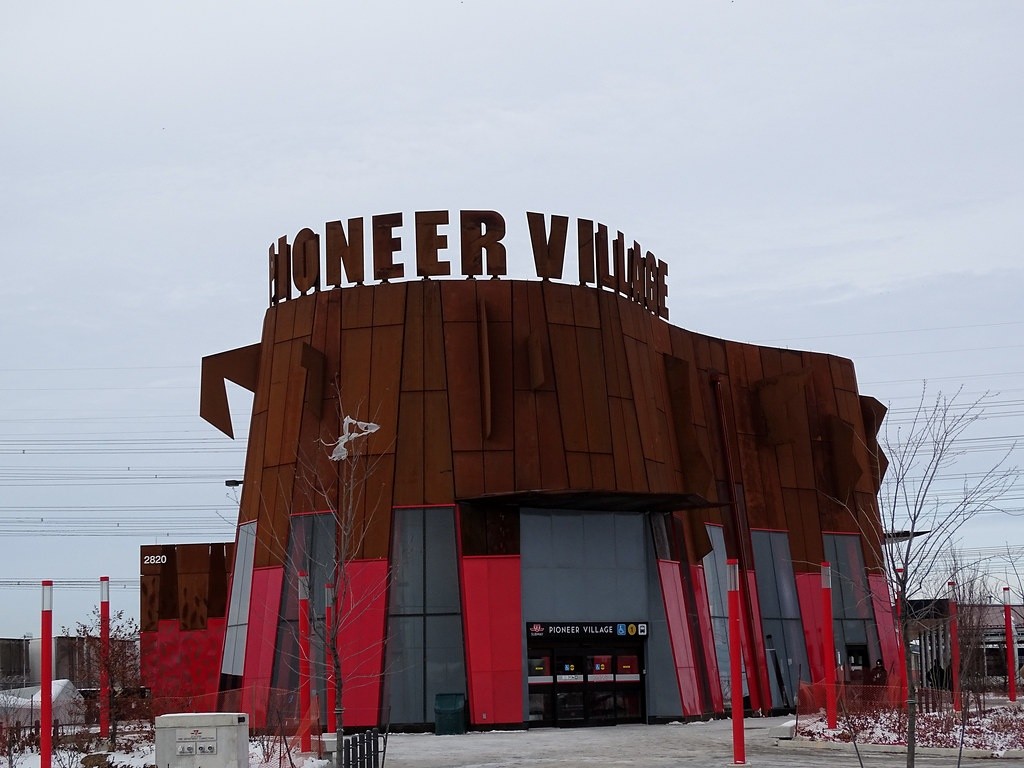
[434,692,465,735]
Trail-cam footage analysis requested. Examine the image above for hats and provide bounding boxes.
[876,659,882,665]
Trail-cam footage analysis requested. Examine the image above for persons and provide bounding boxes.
[945,658,955,704]
[868,659,887,704]
[927,658,944,712]
